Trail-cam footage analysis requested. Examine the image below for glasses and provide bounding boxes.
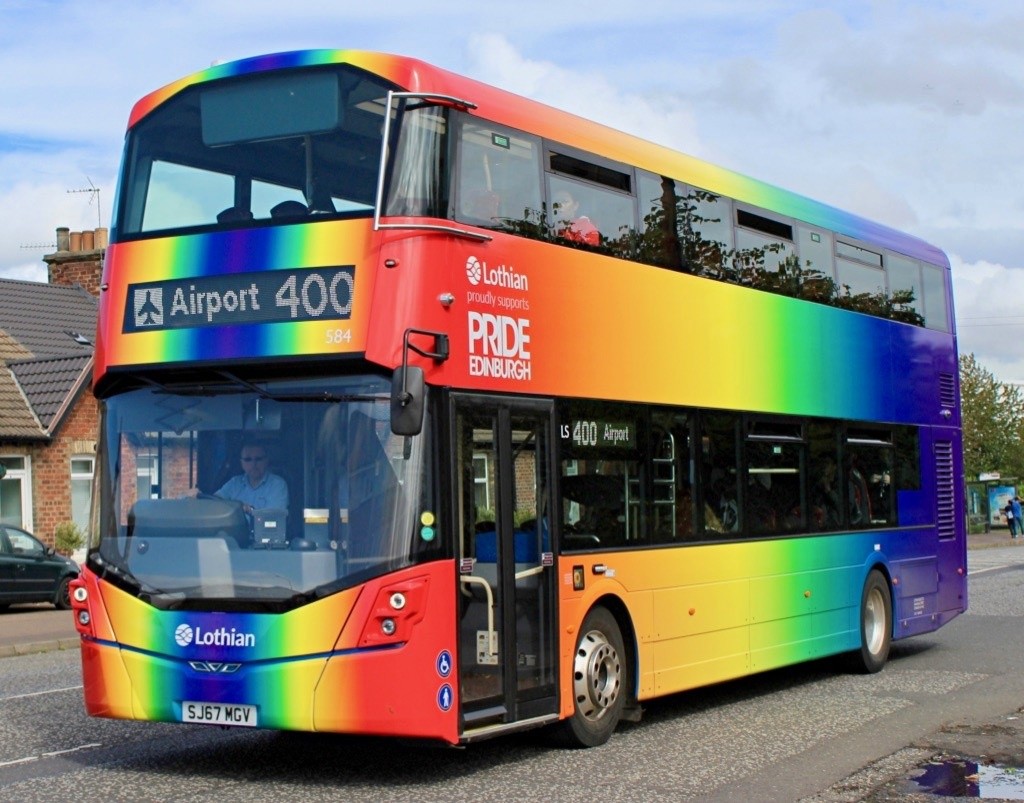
[242,455,266,462]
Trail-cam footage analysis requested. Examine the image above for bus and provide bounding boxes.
[63,50,968,751]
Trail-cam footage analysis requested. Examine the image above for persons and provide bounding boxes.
[575,451,860,540]
[1005,496,1024,538]
[556,190,600,248]
[186,441,288,544]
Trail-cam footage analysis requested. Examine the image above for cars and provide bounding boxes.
[0,524,81,613]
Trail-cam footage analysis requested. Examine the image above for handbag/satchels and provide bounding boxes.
[1006,511,1014,519]
[704,502,726,534]
